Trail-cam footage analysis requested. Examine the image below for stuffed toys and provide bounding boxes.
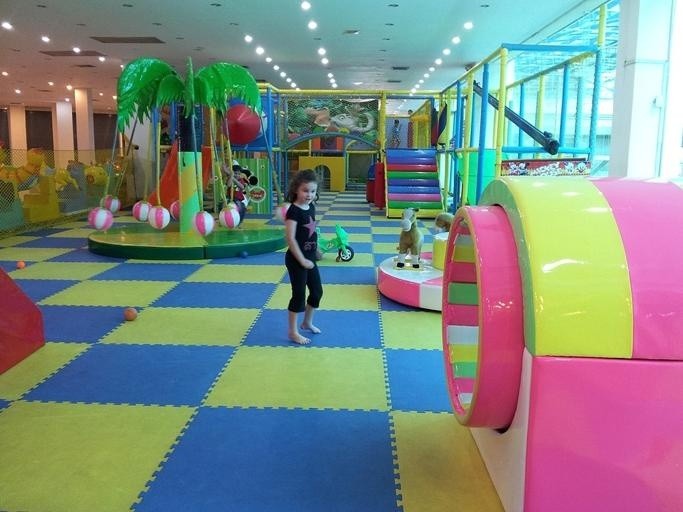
[397,208,424,268]
[435,213,454,232]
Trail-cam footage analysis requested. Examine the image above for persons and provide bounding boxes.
[285,169,323,344]
[392,120,402,149]
[219,165,246,229]
[408,110,414,115]
